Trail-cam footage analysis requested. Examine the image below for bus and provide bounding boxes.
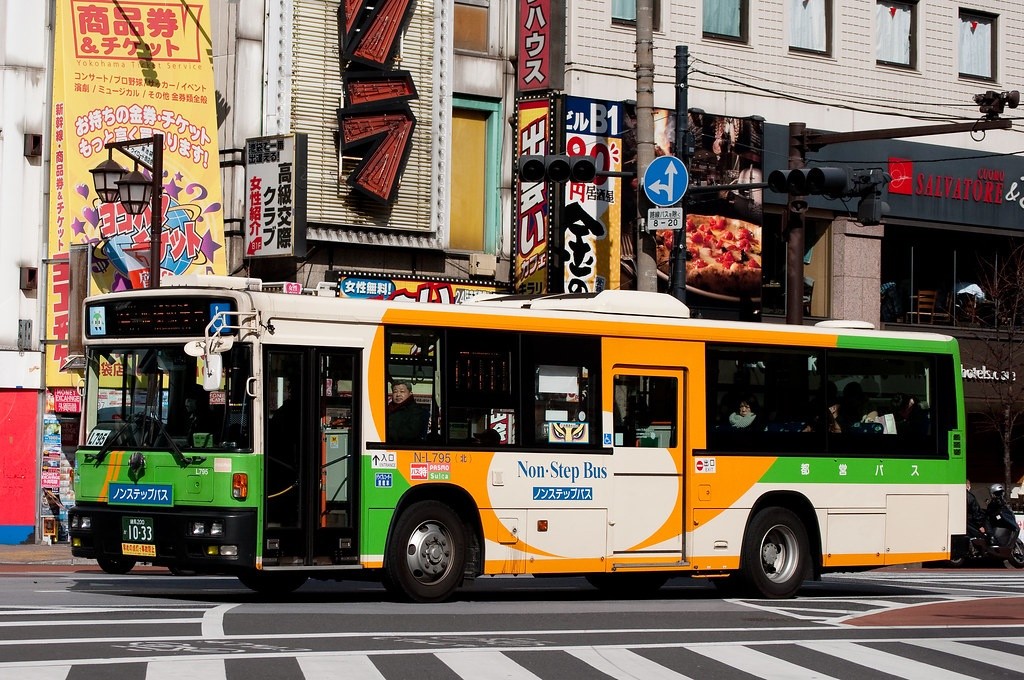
[67,285,967,599]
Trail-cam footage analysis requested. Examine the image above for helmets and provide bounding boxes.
[988,484,1005,498]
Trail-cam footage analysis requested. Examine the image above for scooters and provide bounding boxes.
[949,483,1024,568]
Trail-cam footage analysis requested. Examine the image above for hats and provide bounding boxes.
[473,428,501,442]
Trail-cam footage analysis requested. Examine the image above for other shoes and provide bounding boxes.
[988,538,1000,548]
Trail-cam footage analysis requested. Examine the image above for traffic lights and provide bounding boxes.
[517,152,596,184]
[767,165,850,198]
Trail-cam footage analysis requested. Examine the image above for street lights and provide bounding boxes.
[88,132,164,289]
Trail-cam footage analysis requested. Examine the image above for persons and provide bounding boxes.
[729,394,768,432]
[387,380,430,442]
[473,429,501,445]
[801,403,842,433]
[183,384,215,436]
[889,392,929,437]
